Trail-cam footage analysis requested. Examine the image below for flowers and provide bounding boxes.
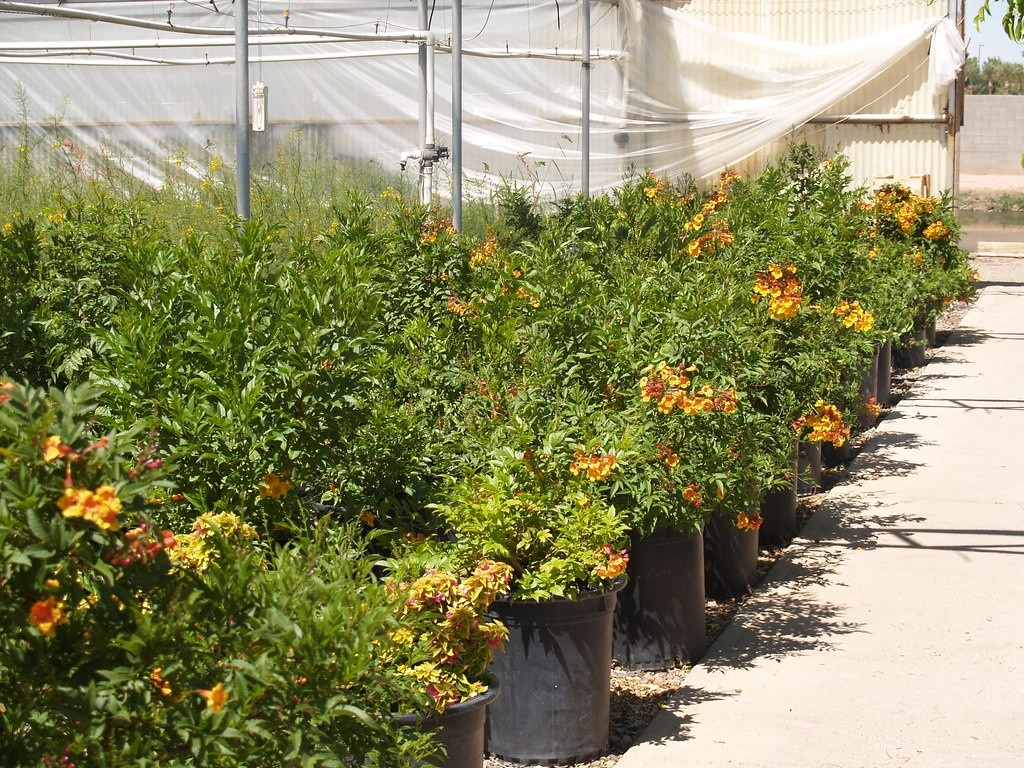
[1,127,985,768]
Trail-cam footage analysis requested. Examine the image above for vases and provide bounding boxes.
[853,342,881,431]
[877,340,892,405]
[377,670,501,768]
[893,323,926,367]
[798,433,823,498]
[483,578,628,763]
[705,490,760,602]
[613,520,705,668]
[820,435,852,465]
[926,316,936,348]
[758,435,800,546]
